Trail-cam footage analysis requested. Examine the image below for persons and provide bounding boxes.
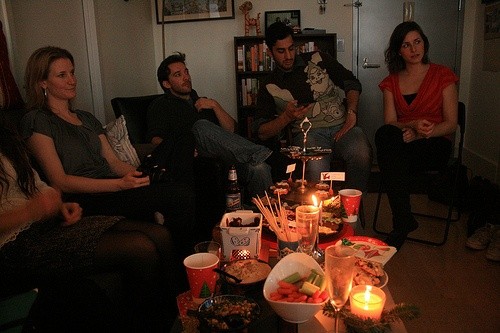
[0,81,179,320]
[148,51,273,194]
[19,47,198,253]
[252,21,372,227]
[375,21,460,253]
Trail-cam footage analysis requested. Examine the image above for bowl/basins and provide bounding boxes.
[220,210,263,260]
[221,259,273,288]
[198,294,260,333]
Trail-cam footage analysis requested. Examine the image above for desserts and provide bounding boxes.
[270,179,330,198]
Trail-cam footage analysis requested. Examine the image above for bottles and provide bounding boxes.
[226,164,242,212]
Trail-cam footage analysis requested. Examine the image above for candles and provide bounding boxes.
[351,285,383,320]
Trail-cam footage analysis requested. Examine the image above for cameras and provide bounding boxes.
[135,158,169,182]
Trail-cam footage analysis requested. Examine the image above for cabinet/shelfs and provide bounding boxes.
[234,33,337,139]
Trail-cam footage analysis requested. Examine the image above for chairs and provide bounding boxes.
[111,94,166,144]
[373,102,466,247]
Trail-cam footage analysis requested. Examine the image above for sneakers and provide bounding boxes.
[466,222,494,250]
[484,225,500,262]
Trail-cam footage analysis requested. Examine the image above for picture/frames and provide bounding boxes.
[265,10,301,35]
[484,3,500,40]
[155,0,235,25]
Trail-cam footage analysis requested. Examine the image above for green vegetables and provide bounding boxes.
[340,238,354,245]
[203,300,256,318]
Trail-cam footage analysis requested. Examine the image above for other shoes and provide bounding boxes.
[385,213,419,251]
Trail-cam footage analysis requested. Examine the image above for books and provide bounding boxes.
[237,40,315,140]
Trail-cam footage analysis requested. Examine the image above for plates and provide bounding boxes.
[263,252,329,323]
[319,217,343,241]
[269,220,295,232]
[372,270,388,289]
[336,236,389,265]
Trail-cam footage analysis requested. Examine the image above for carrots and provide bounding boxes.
[269,275,328,304]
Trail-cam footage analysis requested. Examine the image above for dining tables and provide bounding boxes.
[206,217,407,333]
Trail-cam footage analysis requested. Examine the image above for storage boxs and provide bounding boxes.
[220,210,262,261]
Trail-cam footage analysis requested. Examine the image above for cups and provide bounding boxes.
[339,189,362,222]
[184,252,219,305]
[350,285,386,323]
[296,205,320,256]
[277,233,298,256]
[195,242,221,281]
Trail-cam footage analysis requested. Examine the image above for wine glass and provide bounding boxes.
[325,244,355,333]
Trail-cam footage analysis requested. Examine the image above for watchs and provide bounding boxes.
[347,109,357,115]
